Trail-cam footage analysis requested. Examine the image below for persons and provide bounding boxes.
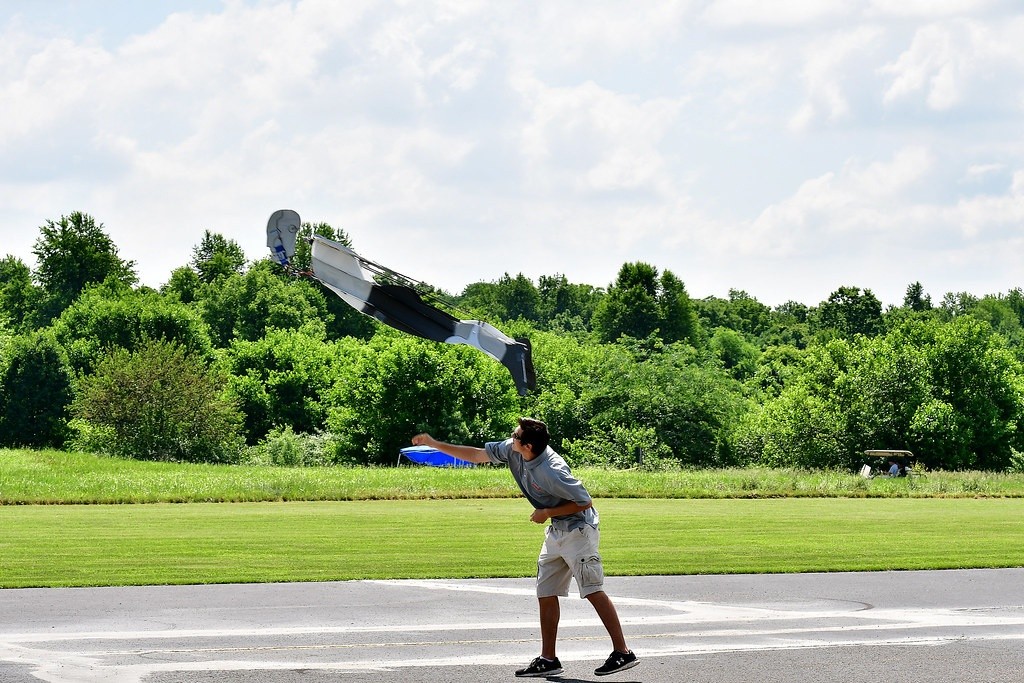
[882,460,898,478]
[412,418,640,676]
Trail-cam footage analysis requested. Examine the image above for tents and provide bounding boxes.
[397,445,478,468]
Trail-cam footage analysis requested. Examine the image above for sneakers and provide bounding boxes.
[594,650,640,676]
[514,657,564,677]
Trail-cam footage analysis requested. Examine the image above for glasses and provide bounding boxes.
[513,432,522,440]
